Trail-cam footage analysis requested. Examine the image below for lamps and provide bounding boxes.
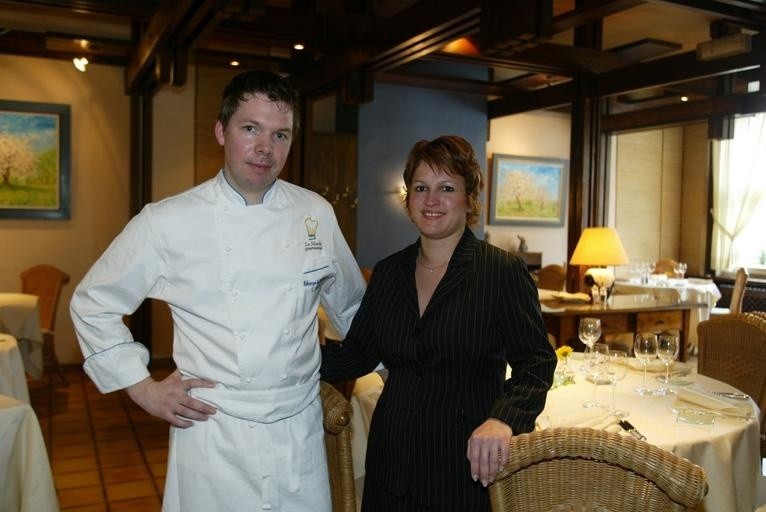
[570,226,631,305]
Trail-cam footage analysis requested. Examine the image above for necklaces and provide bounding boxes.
[415,255,447,274]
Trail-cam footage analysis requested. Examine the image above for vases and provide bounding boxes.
[554,364,574,386]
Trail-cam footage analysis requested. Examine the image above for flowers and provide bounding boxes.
[555,345,573,363]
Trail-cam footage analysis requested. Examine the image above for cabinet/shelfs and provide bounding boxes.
[543,306,708,362]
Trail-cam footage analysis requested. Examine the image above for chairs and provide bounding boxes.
[21,264,71,390]
[320,379,356,512]
[729,266,749,312]
[482,427,707,512]
[696,313,766,465]
[537,265,569,291]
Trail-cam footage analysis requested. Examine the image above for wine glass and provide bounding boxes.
[634,262,656,285]
[633,332,657,397]
[656,334,680,397]
[582,343,610,409]
[673,261,687,286]
[603,349,629,419]
[576,316,602,373]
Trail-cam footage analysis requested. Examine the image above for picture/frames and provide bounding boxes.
[1,99,73,221]
[489,154,569,230]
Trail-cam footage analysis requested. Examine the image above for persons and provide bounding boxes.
[69,70,369,512]
[321,134,557,512]
